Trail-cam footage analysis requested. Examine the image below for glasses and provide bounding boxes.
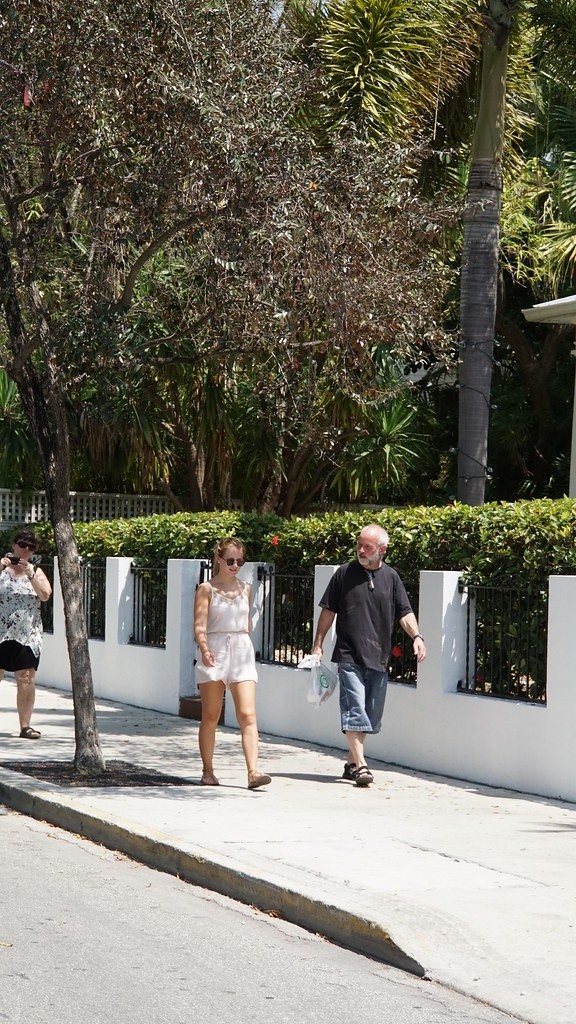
[217,554,245,567]
[15,541,36,551]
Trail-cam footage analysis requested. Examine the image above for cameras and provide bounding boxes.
[7,556,21,565]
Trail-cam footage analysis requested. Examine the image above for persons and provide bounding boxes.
[313,524,428,785]
[0,531,52,739]
[193,537,272,789]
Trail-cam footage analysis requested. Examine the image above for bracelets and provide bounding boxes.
[29,574,34,580]
[413,634,424,641]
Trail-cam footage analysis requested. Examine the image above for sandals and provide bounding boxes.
[200,768,219,785]
[355,766,373,785]
[247,770,271,789]
[342,763,356,781]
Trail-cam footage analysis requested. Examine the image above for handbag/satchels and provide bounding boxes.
[297,654,338,707]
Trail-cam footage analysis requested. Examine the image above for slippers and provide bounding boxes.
[20,727,41,738]
